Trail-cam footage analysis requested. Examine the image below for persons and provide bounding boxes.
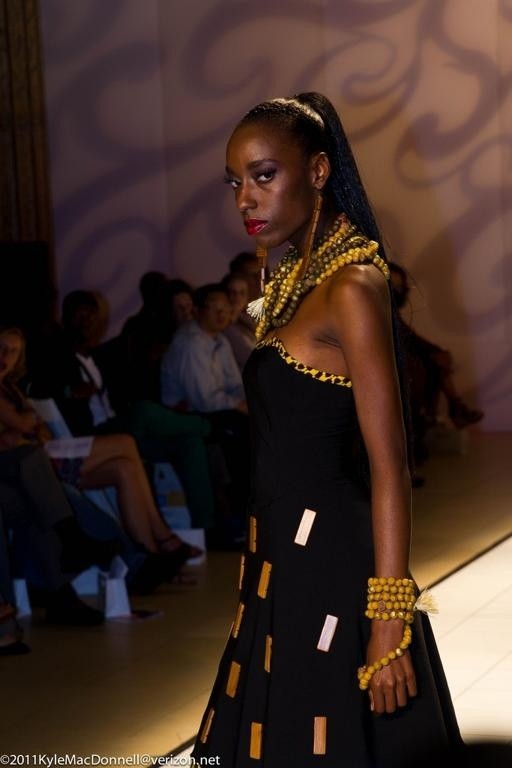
[184,88,465,767]
[0,250,486,656]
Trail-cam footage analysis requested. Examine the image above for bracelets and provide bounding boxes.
[356,572,418,693]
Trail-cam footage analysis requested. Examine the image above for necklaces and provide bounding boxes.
[244,209,392,341]
